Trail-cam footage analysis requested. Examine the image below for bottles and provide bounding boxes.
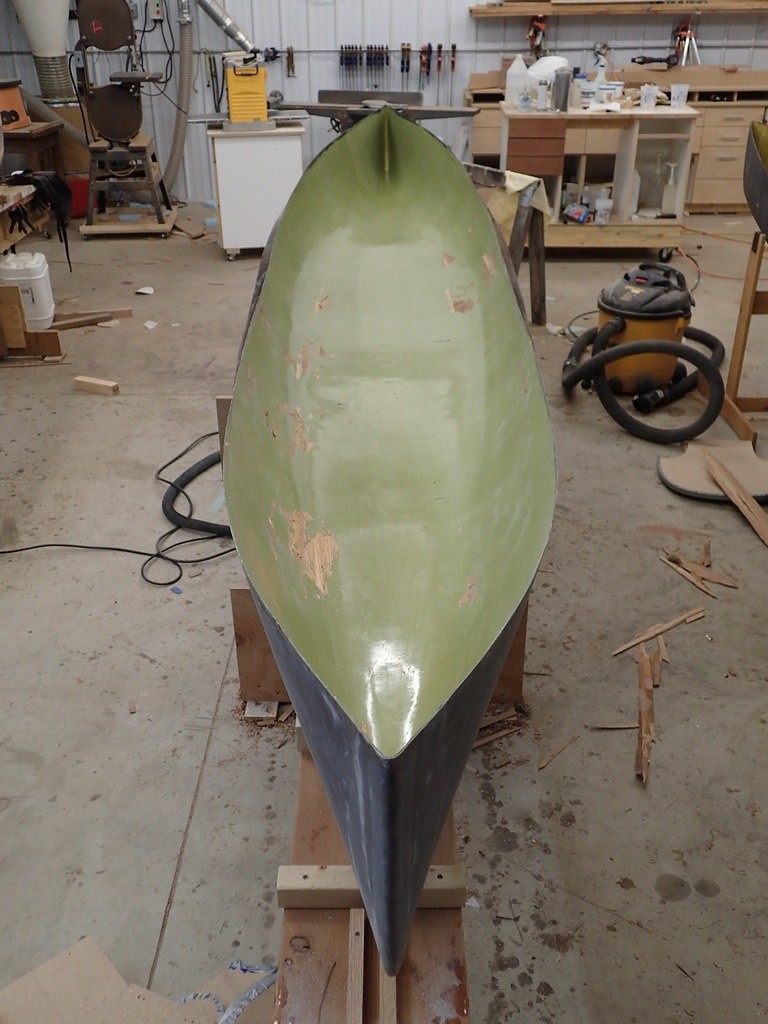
[506,54,529,109]
[573,74,586,109]
[594,65,606,101]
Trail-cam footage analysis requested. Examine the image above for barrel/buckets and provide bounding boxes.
[0,252,54,329]
[66,178,95,218]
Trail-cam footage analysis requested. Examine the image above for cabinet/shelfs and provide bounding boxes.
[463,84,768,215]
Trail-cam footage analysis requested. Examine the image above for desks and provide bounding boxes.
[5,119,65,175]
[499,101,701,222]
[0,184,49,256]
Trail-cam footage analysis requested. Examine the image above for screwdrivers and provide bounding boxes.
[339,44,457,108]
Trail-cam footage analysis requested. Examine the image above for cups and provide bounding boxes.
[599,85,616,102]
[641,87,658,110]
[537,80,547,109]
[671,85,688,109]
[596,198,613,224]
[607,82,625,98]
[564,183,577,206]
